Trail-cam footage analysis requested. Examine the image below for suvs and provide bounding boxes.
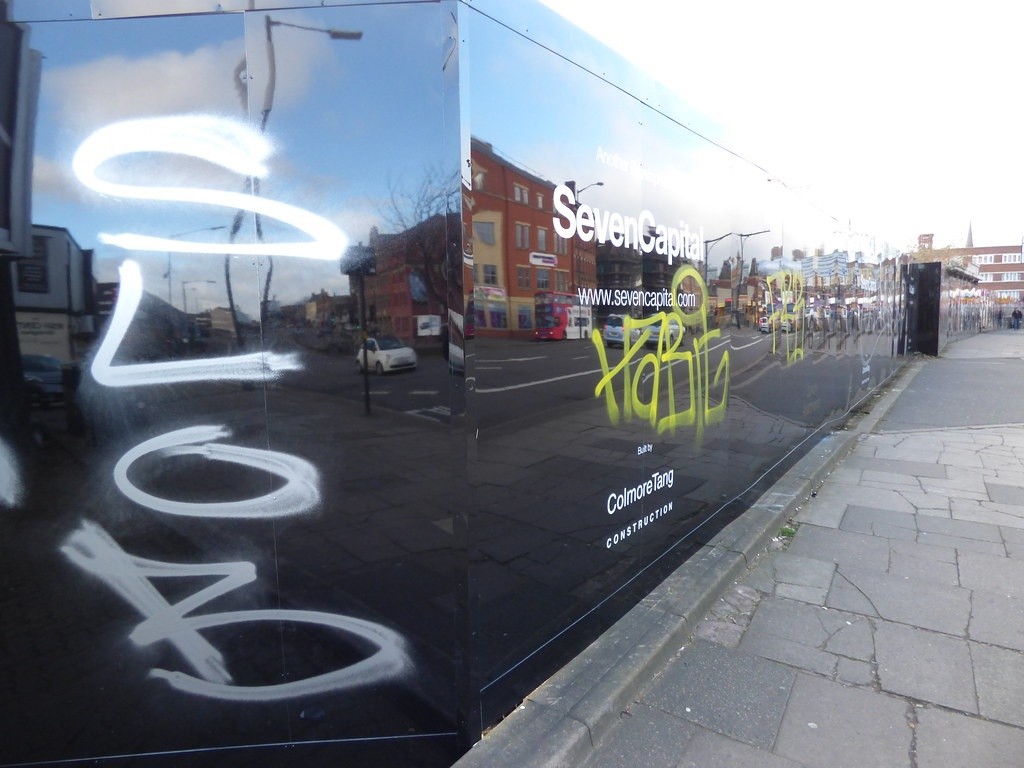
[645,313,686,349]
[604,314,641,348]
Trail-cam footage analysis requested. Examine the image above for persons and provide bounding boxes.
[1012,308,1022,330]
[998,309,1003,329]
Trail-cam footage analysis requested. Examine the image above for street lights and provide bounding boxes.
[253,14,360,355]
[575,181,603,293]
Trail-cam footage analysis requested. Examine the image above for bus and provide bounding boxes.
[532,290,593,339]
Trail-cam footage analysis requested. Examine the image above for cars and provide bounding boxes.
[354,336,419,376]
[781,322,792,333]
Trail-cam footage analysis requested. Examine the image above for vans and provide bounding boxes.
[761,317,769,332]
